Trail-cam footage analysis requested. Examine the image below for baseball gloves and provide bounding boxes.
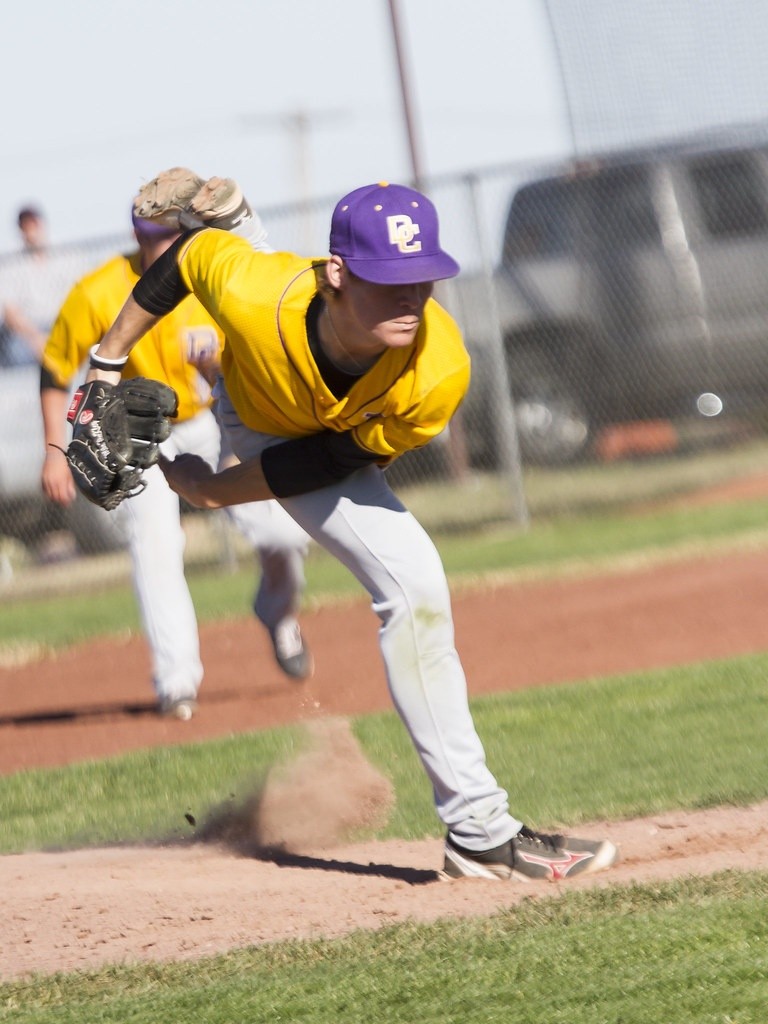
[66,376,180,511]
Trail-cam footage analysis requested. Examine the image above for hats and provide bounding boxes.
[328,181,460,285]
[133,192,195,242]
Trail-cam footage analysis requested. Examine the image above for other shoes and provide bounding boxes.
[163,697,198,721]
[254,604,314,683]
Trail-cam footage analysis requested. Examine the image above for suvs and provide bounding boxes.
[493,126,768,465]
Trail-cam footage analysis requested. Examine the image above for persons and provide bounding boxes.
[66,180,619,887]
[0,208,74,367]
[39,168,316,722]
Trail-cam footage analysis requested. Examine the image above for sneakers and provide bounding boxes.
[437,823,617,883]
